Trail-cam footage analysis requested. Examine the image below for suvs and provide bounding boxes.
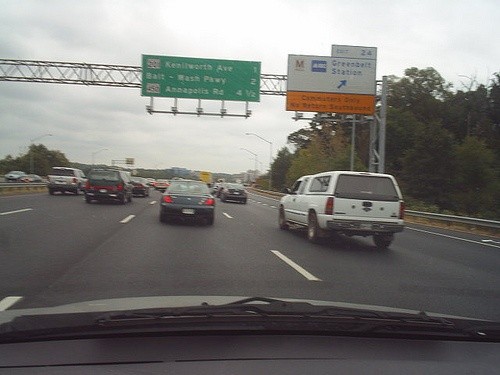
[85,169,133,205]
[47,166,88,195]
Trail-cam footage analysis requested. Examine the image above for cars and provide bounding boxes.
[217,184,225,199]
[147,178,169,192]
[129,179,148,196]
[159,180,215,225]
[211,183,221,194]
[221,184,248,204]
[4,171,25,184]
[20,174,44,184]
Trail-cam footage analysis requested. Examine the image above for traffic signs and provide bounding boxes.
[287,55,375,114]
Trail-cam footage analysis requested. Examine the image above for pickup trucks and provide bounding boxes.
[279,171,406,248]
